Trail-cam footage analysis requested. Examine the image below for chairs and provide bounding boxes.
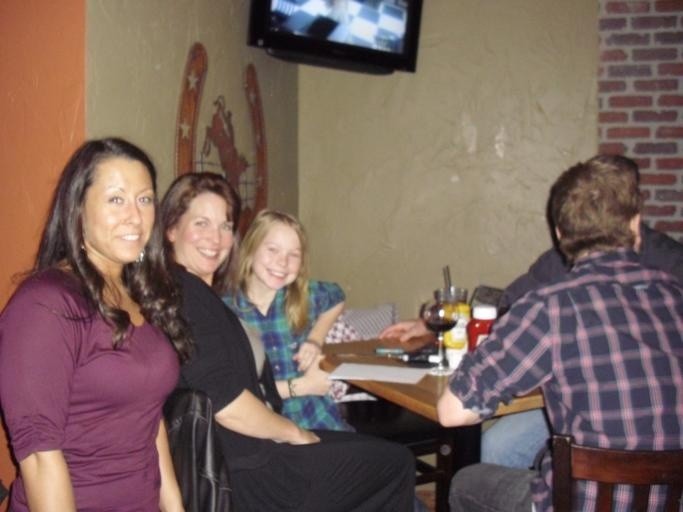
[550,434,683,512]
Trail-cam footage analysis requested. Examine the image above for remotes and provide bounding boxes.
[400,352,436,365]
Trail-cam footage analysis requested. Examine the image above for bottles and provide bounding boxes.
[468,306,500,350]
[385,344,438,367]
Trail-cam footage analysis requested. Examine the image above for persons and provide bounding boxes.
[155,172,418,511]
[215,209,431,512]
[436,160,682,512]
[379,153,682,473]
[0,138,196,512]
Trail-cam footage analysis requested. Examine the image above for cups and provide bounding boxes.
[436,288,468,305]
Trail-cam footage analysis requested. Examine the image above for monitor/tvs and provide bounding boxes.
[247,0,422,77]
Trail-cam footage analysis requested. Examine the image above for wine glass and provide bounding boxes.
[423,299,456,376]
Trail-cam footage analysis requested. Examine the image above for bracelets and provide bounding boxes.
[302,337,325,352]
[287,377,294,399]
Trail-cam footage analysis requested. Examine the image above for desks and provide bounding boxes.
[319,337,545,511]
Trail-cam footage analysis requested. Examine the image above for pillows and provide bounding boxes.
[323,303,397,401]
